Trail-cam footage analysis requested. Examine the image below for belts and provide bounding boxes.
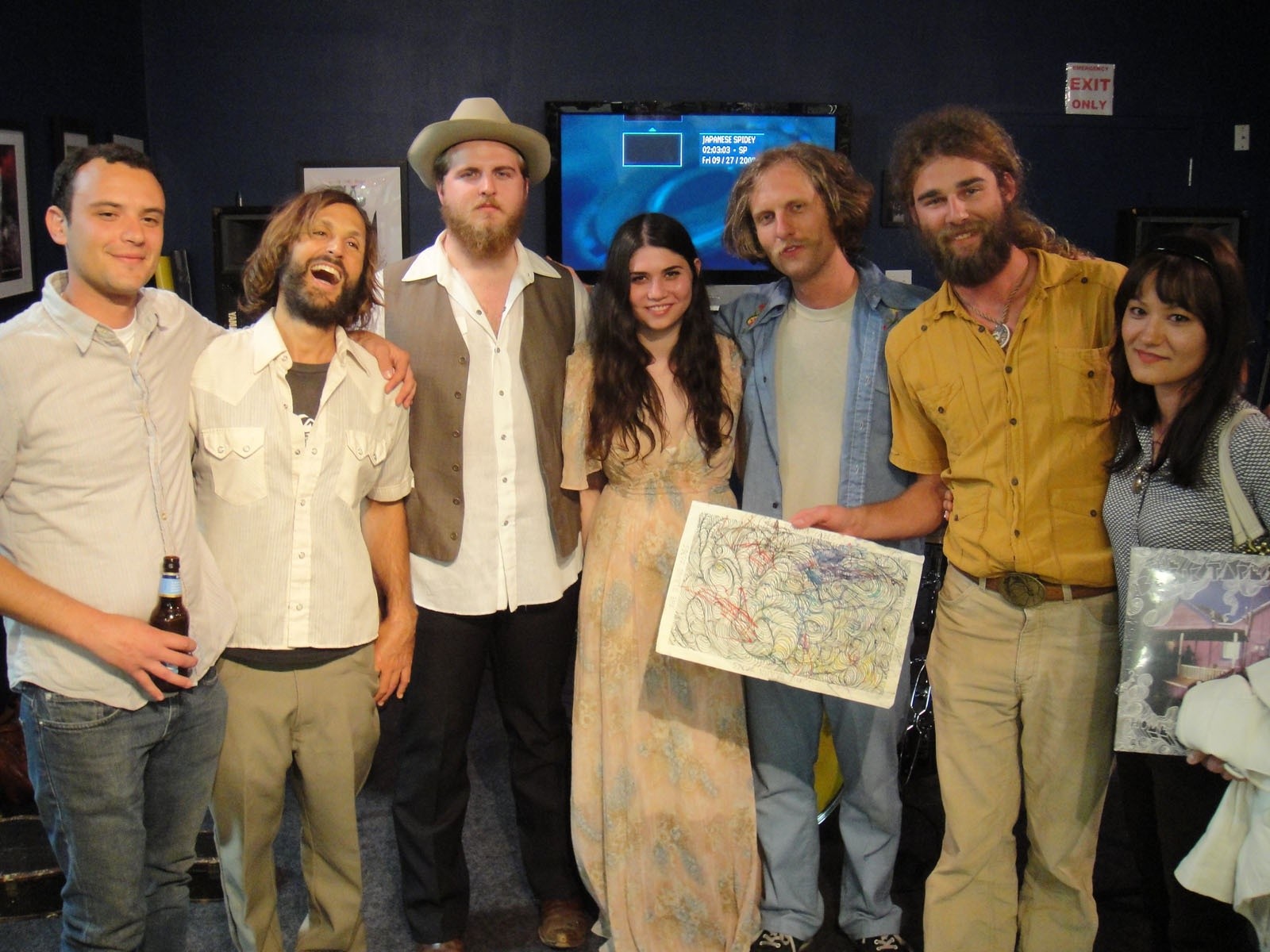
[948,560,1118,607]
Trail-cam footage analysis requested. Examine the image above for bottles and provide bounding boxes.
[149,556,190,693]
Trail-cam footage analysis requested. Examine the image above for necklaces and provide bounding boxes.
[951,248,1031,350]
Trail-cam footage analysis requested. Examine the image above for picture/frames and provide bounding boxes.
[110,132,146,157]
[298,158,412,260]
[0,128,34,300]
[61,131,91,163]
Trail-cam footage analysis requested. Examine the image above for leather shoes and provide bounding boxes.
[419,938,466,952]
[537,896,586,947]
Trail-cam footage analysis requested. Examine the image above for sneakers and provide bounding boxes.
[750,929,814,952]
[855,934,908,952]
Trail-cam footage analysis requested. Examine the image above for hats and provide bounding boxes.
[407,97,551,191]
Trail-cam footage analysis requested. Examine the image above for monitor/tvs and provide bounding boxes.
[545,100,851,288]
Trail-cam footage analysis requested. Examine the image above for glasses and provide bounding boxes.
[1137,235,1222,289]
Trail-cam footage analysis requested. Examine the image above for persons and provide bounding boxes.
[786,107,1136,952]
[561,214,763,952]
[700,144,936,952]
[352,96,599,952]
[188,187,419,952]
[943,227,1270,952]
[0,142,418,952]
